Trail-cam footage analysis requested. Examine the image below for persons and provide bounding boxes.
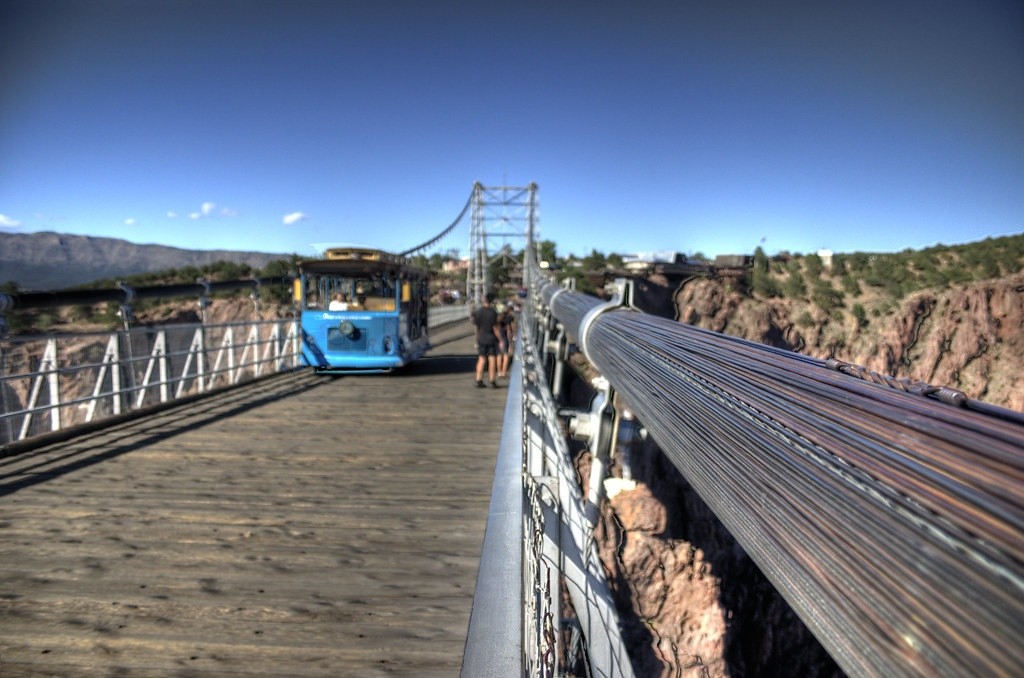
[470,293,516,389]
[329,293,348,311]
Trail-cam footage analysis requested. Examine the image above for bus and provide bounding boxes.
[293,247,431,376]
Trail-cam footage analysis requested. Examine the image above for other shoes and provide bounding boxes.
[475,381,487,388]
[489,382,496,388]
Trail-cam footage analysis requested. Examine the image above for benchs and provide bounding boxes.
[348,297,396,311]
